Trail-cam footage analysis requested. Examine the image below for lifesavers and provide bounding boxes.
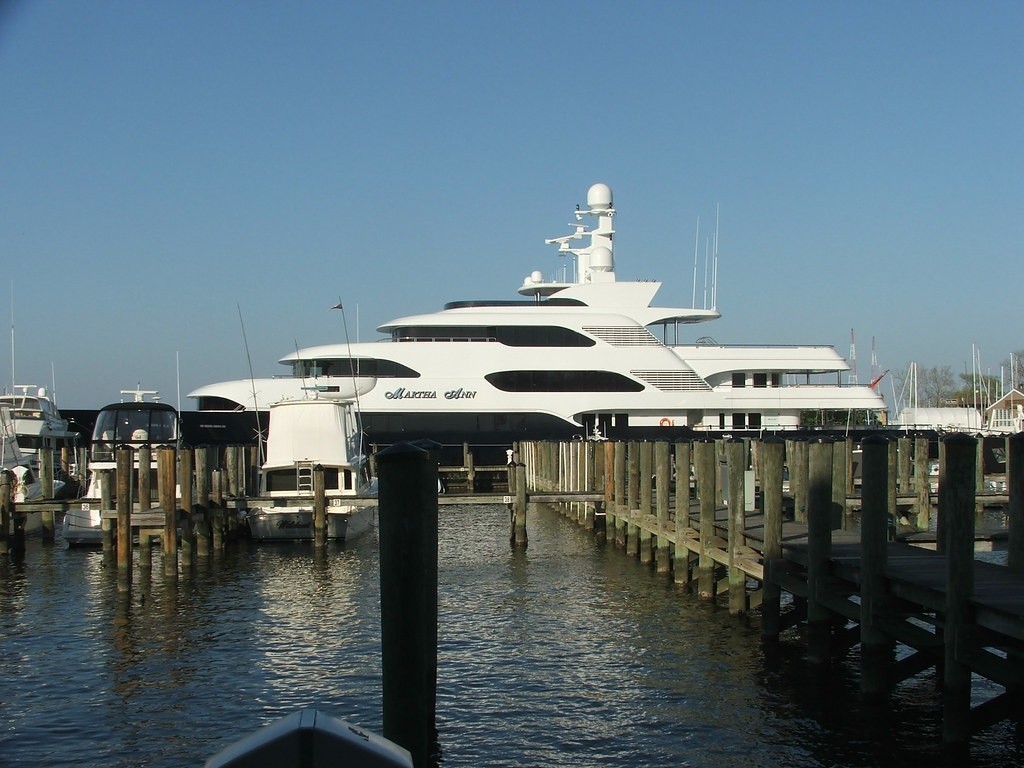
[659,417,671,427]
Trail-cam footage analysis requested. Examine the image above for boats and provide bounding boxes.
[0,281,80,535]
[61,382,187,545]
[241,385,377,540]
[191,183,890,503]
